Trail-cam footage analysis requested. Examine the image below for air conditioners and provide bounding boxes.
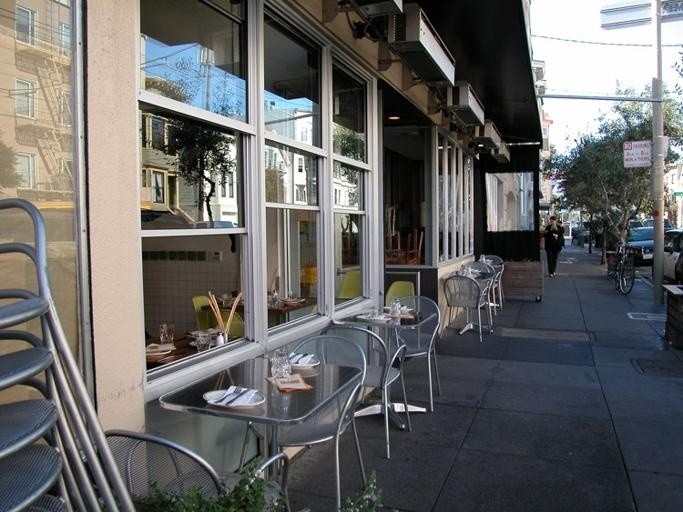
[475,120,501,148]
[491,140,510,163]
[352,0,404,19]
[447,83,485,128]
[388,1,454,87]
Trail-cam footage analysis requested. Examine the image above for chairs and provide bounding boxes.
[444,276,491,342]
[103,428,290,510]
[144,259,362,368]
[0,199,135,511]
[373,295,442,413]
[461,254,505,324]
[318,327,412,458]
[238,334,367,511]
[385,281,416,308]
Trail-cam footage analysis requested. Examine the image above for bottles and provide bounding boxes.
[272,289,278,299]
[216,332,223,346]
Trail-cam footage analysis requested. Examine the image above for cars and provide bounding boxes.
[572,220,590,241]
[625,219,683,285]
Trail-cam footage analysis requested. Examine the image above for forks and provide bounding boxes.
[213,383,242,403]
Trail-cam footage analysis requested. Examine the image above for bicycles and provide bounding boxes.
[607,242,634,295]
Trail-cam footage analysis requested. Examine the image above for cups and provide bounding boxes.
[465,263,472,273]
[159,324,174,343]
[194,332,210,353]
[271,348,292,378]
[389,298,401,316]
[480,254,486,262]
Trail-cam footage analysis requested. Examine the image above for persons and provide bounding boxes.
[544,216,562,278]
[558,220,564,234]
[556,220,564,252]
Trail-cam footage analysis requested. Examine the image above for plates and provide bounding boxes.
[284,352,320,370]
[146,348,171,356]
[355,314,391,323]
[187,329,221,336]
[383,305,412,314]
[201,389,265,409]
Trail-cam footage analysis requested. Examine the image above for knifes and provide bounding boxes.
[225,388,248,405]
